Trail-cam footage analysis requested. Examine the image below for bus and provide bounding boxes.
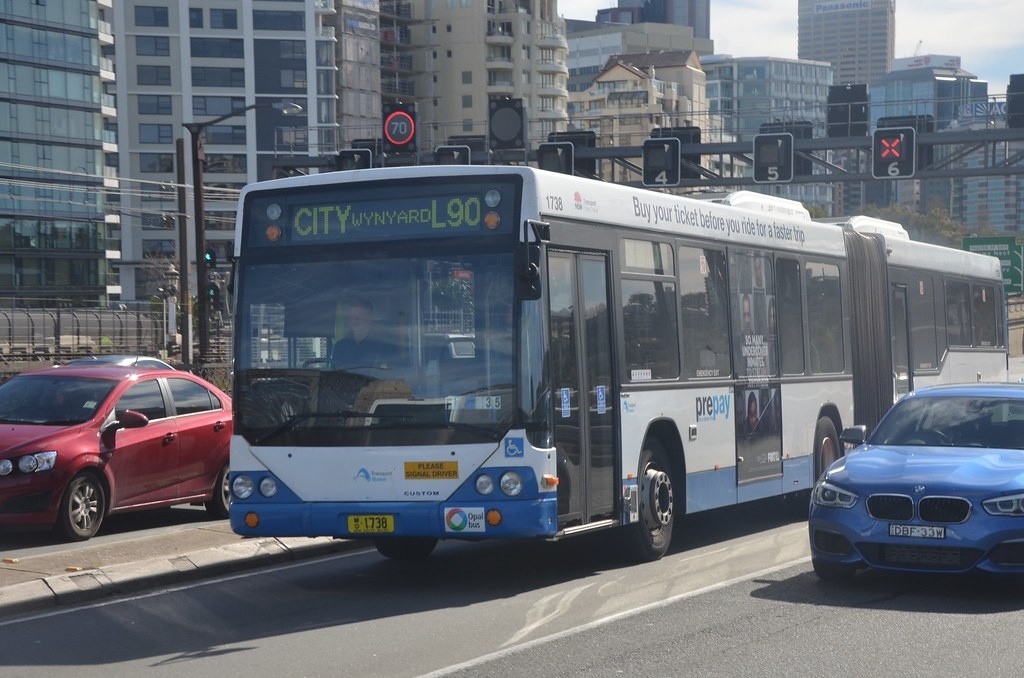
[220,165,1012,572]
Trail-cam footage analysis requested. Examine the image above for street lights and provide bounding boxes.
[180,101,303,380]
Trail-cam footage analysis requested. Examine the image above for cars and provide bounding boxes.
[54,354,178,372]
[0,365,236,544]
[805,380,1024,592]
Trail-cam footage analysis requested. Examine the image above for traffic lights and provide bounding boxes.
[333,148,373,174]
[202,247,218,268]
[206,280,220,299]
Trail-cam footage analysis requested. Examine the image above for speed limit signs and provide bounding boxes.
[383,111,415,145]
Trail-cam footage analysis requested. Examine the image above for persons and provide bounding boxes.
[331,298,400,371]
[753,251,765,288]
[744,391,760,433]
[741,295,754,341]
[762,390,770,429]
[768,298,779,345]
